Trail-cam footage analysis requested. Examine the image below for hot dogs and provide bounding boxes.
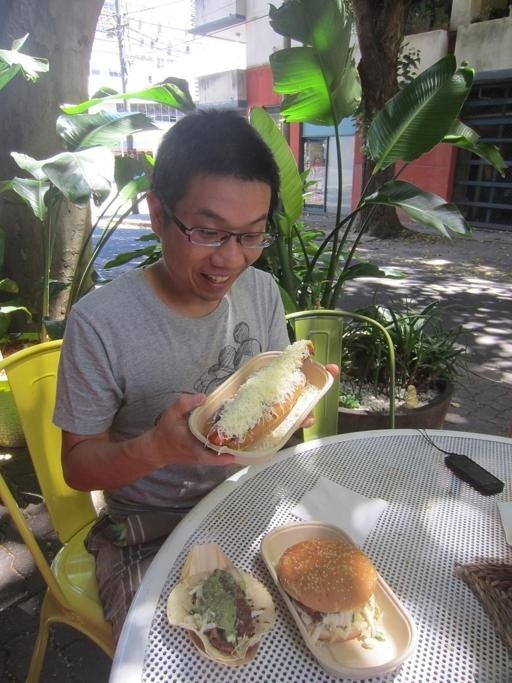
[209,339,316,451]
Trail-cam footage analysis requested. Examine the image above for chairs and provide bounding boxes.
[276,308,399,444]
[0,338,121,682]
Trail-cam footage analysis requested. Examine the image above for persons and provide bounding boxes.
[52,111,341,662]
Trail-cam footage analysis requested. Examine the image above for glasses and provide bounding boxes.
[159,199,279,249]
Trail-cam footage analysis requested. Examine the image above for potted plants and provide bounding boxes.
[300,296,467,438]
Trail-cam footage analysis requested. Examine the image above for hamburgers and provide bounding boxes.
[277,537,391,649]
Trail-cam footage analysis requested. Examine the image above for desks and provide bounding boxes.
[103,424,512,683]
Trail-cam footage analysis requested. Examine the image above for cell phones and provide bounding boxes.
[445,453,504,496]
